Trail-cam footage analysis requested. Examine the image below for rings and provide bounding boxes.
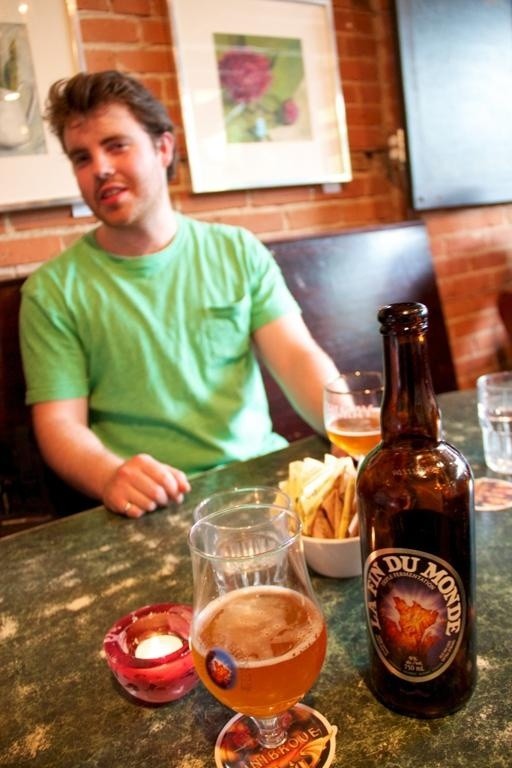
[117,501,137,518]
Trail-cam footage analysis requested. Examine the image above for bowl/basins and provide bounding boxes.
[106,600,197,708]
[270,499,363,580]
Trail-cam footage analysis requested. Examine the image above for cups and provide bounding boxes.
[475,371,512,473]
[323,373,384,459]
[196,484,293,597]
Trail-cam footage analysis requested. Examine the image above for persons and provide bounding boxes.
[19,72,358,519]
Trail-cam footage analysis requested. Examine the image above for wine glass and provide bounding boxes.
[185,512,331,766]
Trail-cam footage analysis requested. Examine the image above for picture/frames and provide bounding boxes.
[167,0,353,197]
[0,0,103,214]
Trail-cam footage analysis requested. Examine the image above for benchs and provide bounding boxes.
[0,221,460,532]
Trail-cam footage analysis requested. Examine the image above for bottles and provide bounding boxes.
[359,300,478,721]
